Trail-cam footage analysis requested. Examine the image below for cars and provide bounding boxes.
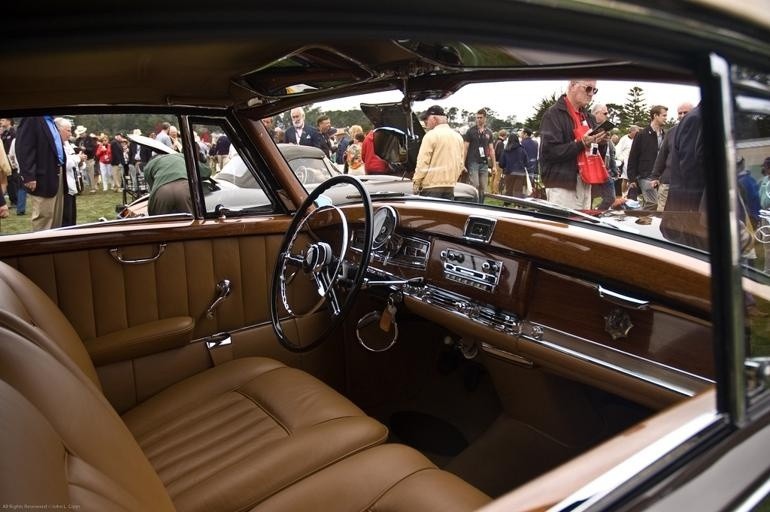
[0,1,770,509]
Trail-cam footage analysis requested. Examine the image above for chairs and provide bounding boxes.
[1,261,391,512]
[2,329,490,512]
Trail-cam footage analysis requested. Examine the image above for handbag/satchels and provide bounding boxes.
[573,120,609,184]
[522,167,532,197]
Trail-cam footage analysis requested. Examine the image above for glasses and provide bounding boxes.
[600,110,609,116]
[580,83,599,94]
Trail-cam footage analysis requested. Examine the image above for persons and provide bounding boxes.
[540,77,608,213]
[262,104,769,234]
[1,112,236,234]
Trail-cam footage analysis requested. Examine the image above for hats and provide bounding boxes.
[74,125,87,135]
[420,105,449,120]
[523,127,533,134]
[122,139,129,144]
[335,128,347,137]
[116,132,125,137]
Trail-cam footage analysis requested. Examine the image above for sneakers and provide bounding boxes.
[746,304,769,319]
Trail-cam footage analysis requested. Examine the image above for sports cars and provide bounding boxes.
[98,130,484,228]
